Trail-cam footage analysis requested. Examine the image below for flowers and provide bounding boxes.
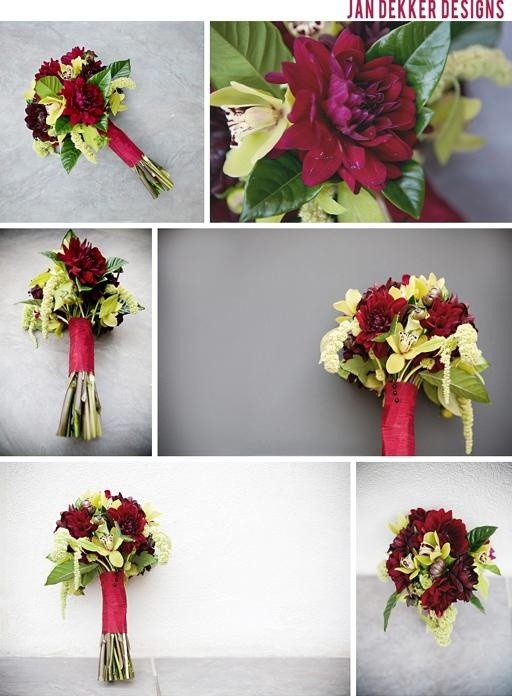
[11,230,144,443]
[319,269,489,457]
[372,503,504,648]
[41,485,172,686]
[20,47,175,202]
